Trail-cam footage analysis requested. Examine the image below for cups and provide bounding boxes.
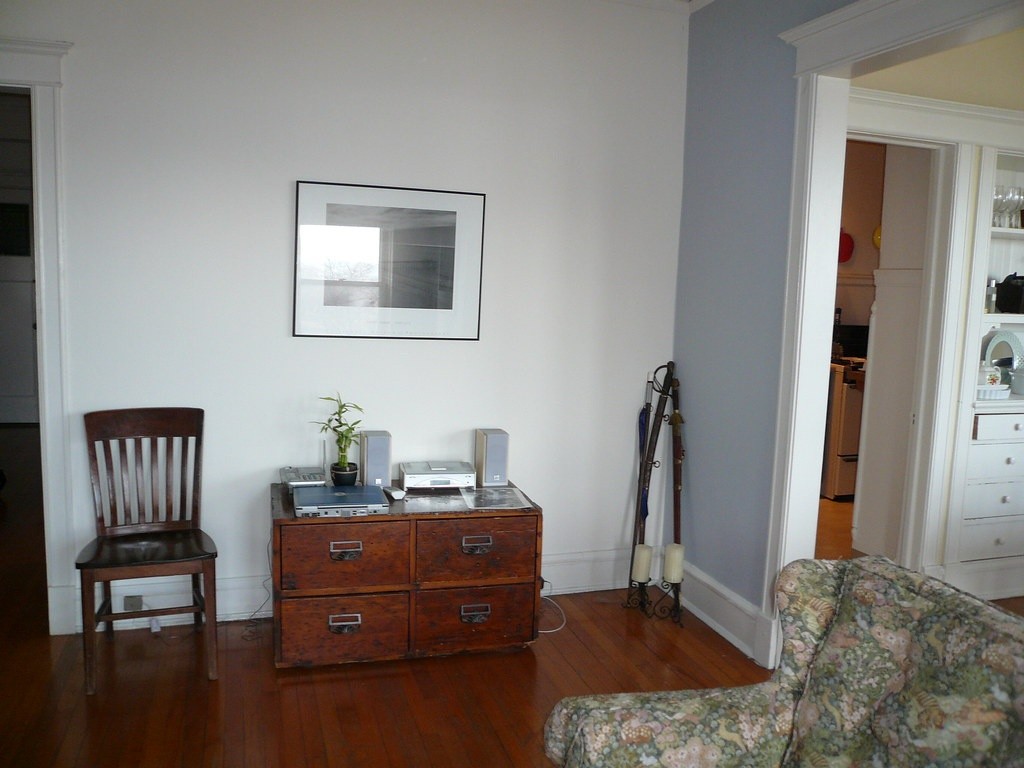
[1009,370,1024,394]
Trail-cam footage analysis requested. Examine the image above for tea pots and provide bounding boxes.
[977,360,1002,385]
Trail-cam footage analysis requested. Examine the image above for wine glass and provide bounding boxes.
[991,184,1024,229]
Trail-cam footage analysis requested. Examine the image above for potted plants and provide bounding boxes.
[307,387,365,488]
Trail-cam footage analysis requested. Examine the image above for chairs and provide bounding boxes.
[73,407,220,697]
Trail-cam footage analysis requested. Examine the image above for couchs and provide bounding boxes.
[544,554,1023,768]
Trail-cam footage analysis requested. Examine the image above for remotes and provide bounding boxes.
[383,487,406,499]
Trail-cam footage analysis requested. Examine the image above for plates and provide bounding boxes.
[977,384,1010,390]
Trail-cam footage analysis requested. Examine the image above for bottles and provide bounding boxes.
[831,307,843,360]
[985,280,998,313]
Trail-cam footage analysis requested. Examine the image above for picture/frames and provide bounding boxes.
[292,180,485,341]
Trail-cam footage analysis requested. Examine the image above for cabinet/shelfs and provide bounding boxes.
[270,479,544,668]
[820,355,868,500]
[940,143,1024,564]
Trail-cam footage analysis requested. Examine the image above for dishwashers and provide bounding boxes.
[822,367,867,498]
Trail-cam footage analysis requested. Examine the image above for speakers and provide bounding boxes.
[475,429,509,486]
[360,431,391,488]
